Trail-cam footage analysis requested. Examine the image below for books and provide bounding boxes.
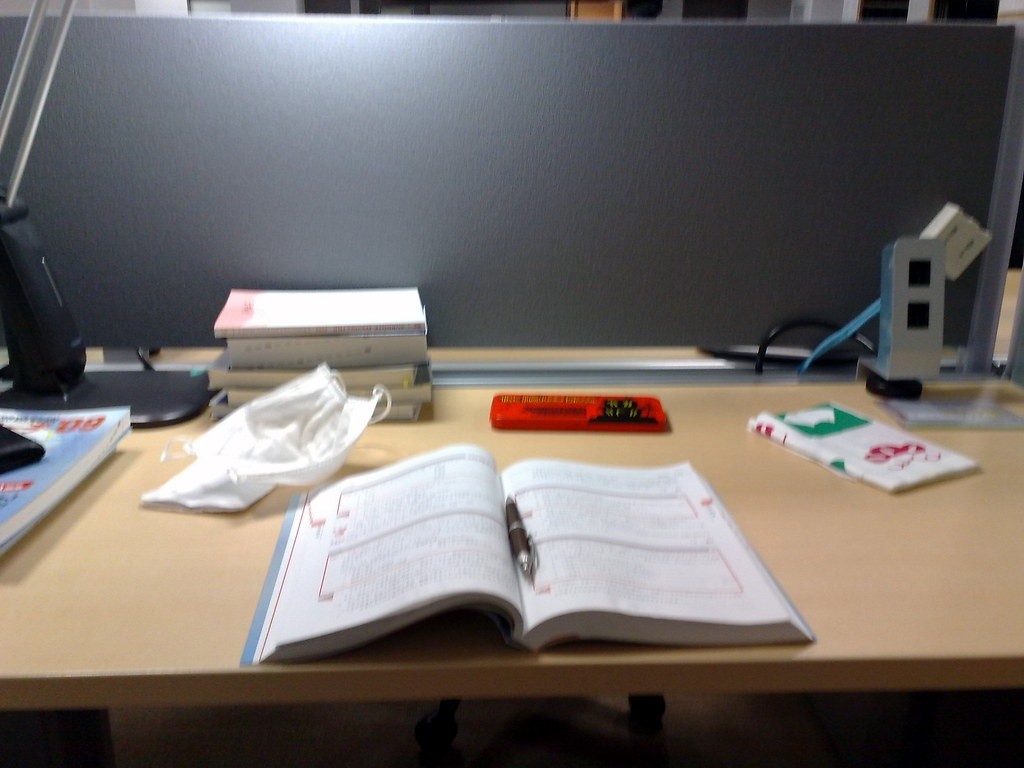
[1,400,132,557]
[205,286,433,423]
[238,444,816,665]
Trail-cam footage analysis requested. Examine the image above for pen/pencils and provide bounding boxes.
[504,495,536,578]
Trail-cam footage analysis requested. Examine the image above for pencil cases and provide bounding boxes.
[490,394,668,433]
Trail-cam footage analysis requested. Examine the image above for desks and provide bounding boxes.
[0,343,1024,768]
